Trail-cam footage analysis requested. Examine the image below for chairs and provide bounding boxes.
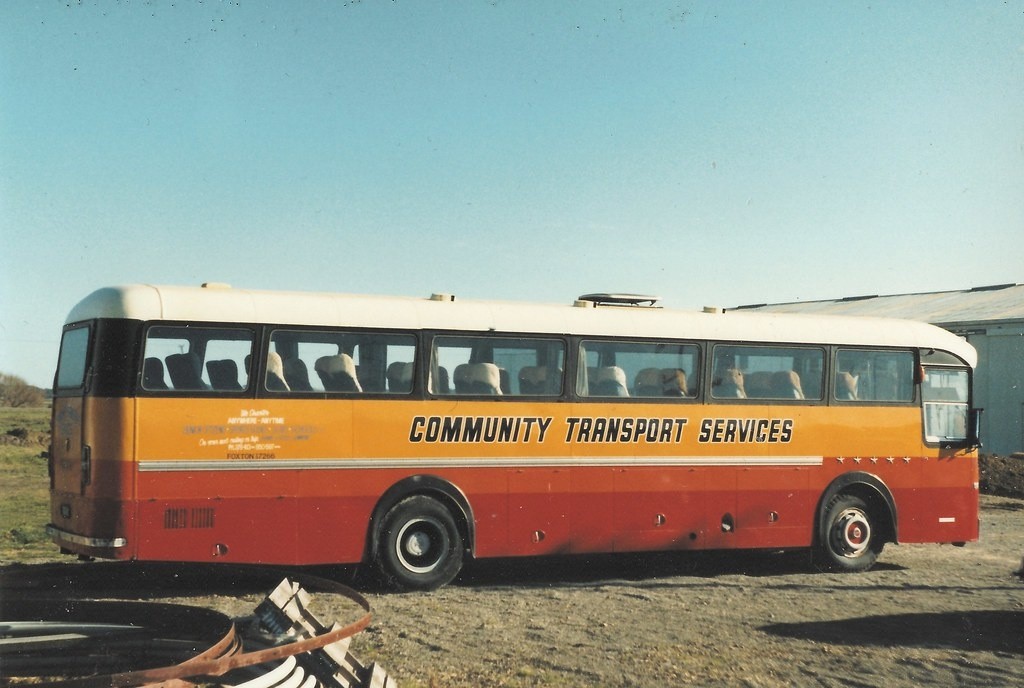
[533,366,562,394]
[354,366,382,392]
[750,372,776,398]
[244,354,251,375]
[713,368,747,398]
[587,367,599,396]
[836,372,859,400]
[496,369,510,394]
[871,371,897,400]
[283,358,313,392]
[773,371,804,398]
[598,366,629,396]
[165,353,208,390]
[743,372,751,398]
[518,366,535,394]
[453,363,477,394]
[659,368,689,397]
[206,359,243,390]
[400,362,414,392]
[687,372,697,396]
[143,357,169,389]
[804,369,821,397]
[472,362,504,395]
[437,367,449,392]
[314,353,363,392]
[634,367,665,396]
[386,361,407,392]
[266,351,291,392]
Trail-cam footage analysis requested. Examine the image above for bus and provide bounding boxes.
[45,279,983,589]
